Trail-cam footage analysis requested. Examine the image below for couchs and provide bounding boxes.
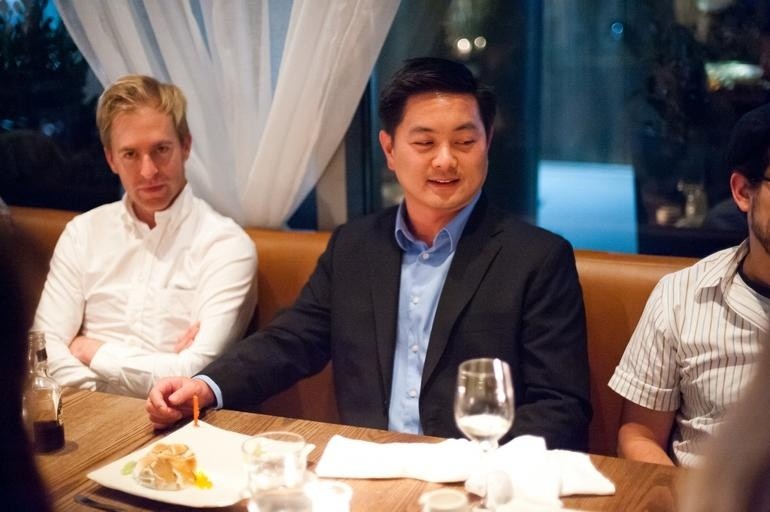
[1,201,770,473]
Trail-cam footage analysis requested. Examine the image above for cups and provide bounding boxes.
[420,489,466,511]
[240,430,355,512]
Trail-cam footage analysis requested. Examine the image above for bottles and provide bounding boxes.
[25,329,67,455]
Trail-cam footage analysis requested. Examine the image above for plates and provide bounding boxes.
[86,418,317,508]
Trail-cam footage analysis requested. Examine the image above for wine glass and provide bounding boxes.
[454,357,515,512]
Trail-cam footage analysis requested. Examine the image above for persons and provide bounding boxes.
[26,76,259,399]
[608,103,770,470]
[0,196,50,512]
[146,58,593,453]
